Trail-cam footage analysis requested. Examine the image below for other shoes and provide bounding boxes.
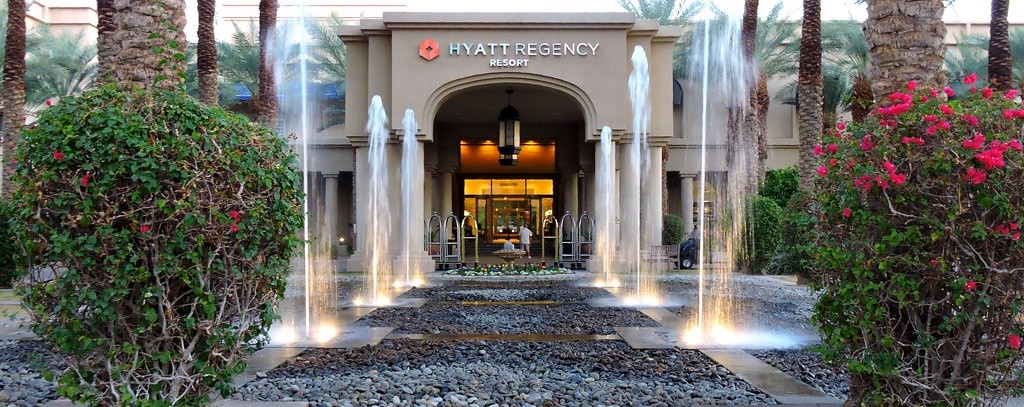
[520,256,522,258]
[528,256,532,258]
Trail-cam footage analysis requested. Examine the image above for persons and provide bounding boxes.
[519,224,532,258]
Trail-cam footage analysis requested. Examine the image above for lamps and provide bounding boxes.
[497,88,521,166]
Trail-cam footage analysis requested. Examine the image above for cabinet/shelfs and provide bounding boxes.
[693,200,716,231]
